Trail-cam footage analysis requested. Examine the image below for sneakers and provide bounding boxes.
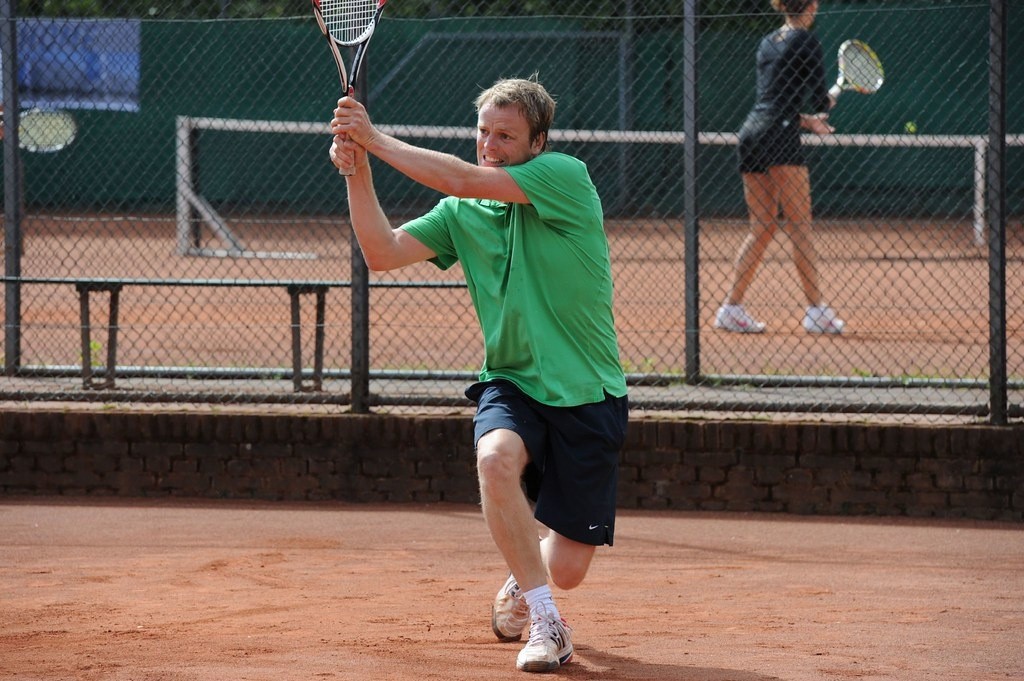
[515,615,574,672]
[804,303,843,335]
[713,301,766,335]
[491,536,542,643]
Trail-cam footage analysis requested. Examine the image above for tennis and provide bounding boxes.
[904,123,917,133]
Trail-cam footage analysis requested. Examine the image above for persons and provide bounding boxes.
[328,79,630,672]
[715,0,843,331]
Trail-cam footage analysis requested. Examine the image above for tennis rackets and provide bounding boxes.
[0,108,77,152]
[309,0,387,176]
[822,40,885,115]
